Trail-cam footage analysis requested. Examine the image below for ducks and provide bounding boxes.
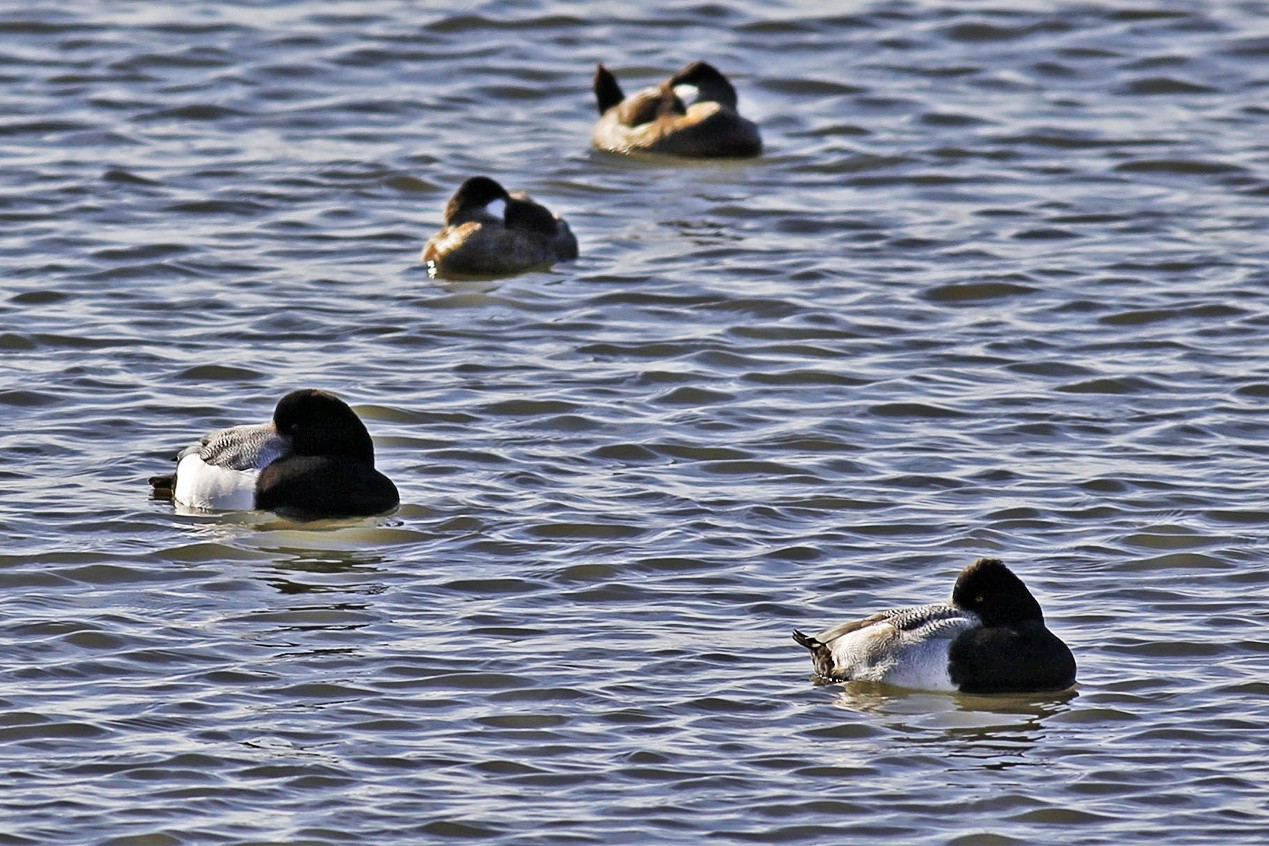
[149,388,400,518]
[421,176,580,279]
[791,558,1077,693]
[595,53,764,159]
